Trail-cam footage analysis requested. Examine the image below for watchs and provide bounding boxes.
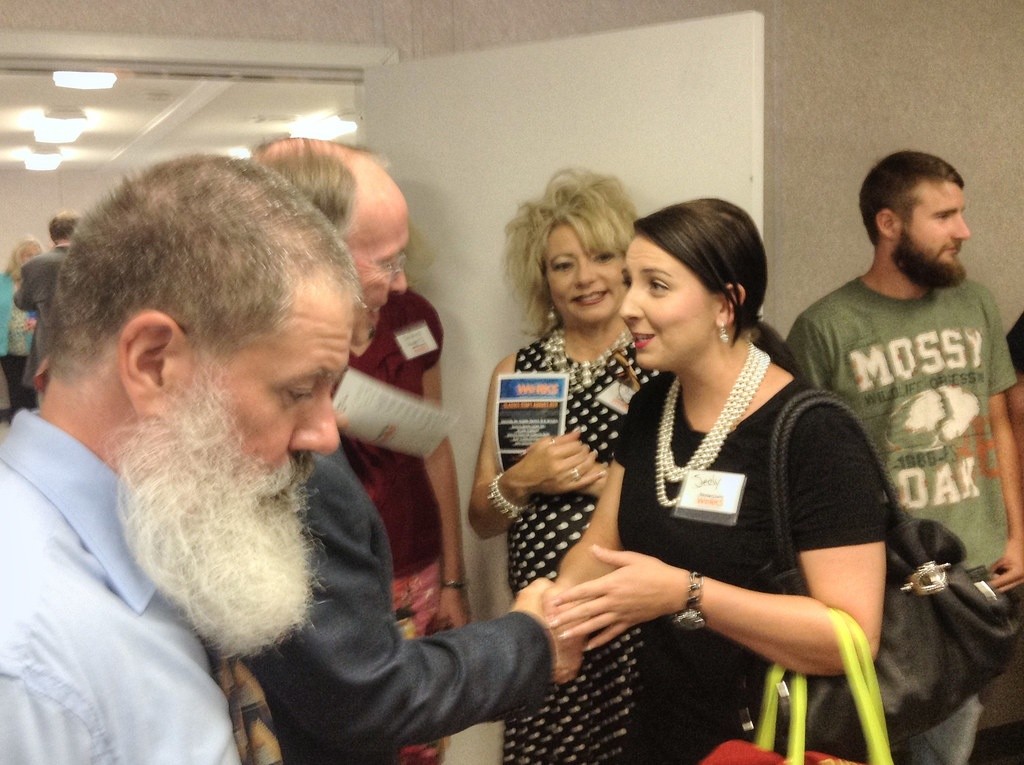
[671,571,706,631]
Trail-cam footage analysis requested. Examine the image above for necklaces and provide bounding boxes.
[541,324,636,394]
[655,342,772,509]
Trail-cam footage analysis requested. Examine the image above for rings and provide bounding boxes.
[570,466,581,480]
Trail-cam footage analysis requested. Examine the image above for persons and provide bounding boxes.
[13,210,83,408]
[787,150,1024,765]
[539,197,894,765]
[467,175,694,765]
[252,137,592,765]
[332,290,470,765]
[0,156,365,765]
[0,237,47,425]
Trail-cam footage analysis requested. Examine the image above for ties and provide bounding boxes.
[199,639,282,765]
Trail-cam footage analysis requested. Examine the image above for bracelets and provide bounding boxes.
[487,471,533,520]
[439,576,466,593]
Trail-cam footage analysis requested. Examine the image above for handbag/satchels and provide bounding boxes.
[732,389,1020,758]
[698,607,894,764]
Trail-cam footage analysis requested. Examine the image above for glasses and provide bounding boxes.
[355,253,407,279]
